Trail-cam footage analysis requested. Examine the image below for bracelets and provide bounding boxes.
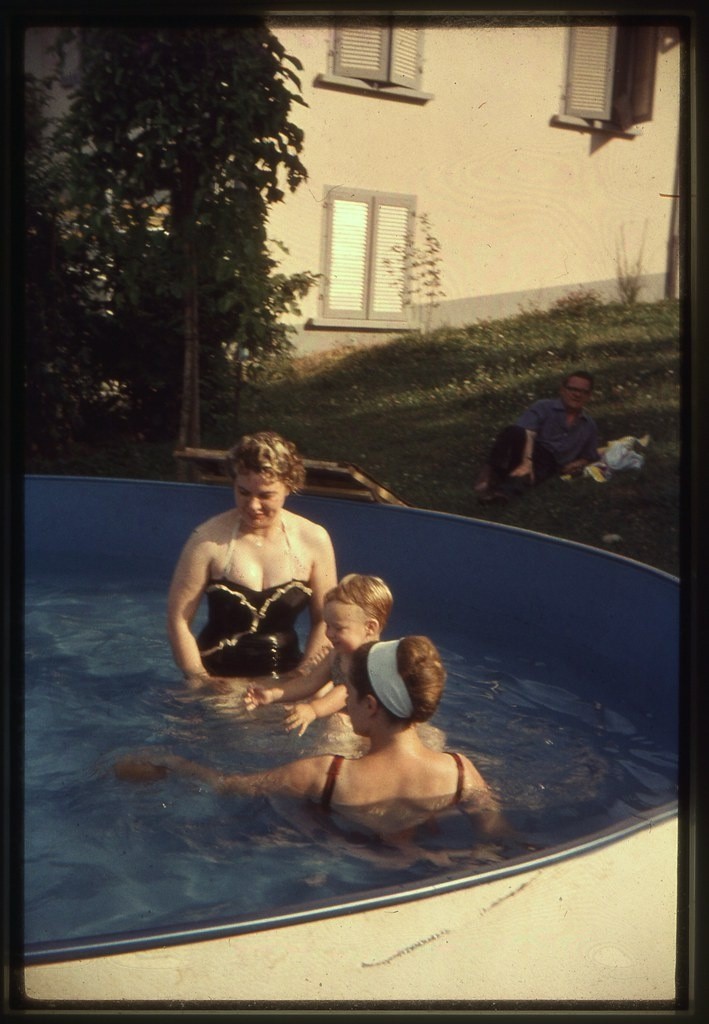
[523,456,534,461]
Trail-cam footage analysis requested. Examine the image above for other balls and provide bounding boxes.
[113,751,168,782]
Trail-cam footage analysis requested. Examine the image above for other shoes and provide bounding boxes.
[479,493,507,505]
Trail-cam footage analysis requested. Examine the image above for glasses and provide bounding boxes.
[566,385,589,395]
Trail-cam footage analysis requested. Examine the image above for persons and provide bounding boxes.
[116,637,504,842]
[472,370,599,505]
[166,434,442,756]
